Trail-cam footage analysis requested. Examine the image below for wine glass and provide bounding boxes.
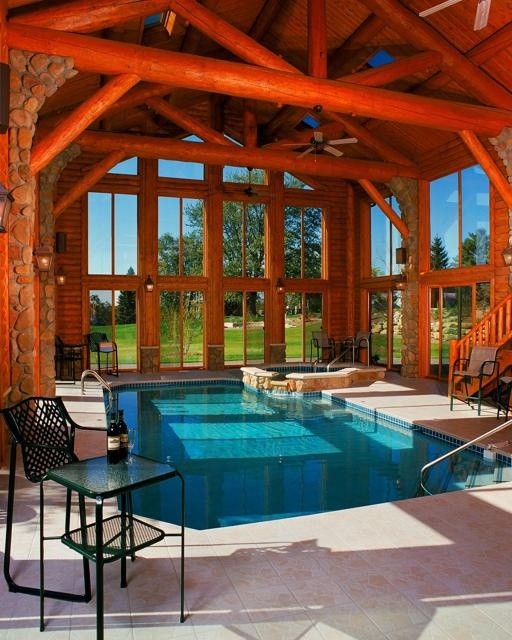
[128,429,135,464]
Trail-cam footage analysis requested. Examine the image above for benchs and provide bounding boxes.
[239,362,386,392]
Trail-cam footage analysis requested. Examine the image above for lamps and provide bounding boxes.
[33,242,53,272]
[395,272,408,290]
[145,275,154,292]
[0,184,15,234]
[54,267,66,286]
[501,247,512,267]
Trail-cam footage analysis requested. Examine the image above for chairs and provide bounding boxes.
[55,332,119,385]
[0,396,137,603]
[449,346,512,422]
[309,330,371,363]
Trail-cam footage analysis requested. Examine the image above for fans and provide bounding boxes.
[419,1,493,33]
[282,132,358,162]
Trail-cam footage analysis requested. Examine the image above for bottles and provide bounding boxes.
[107,410,127,464]
